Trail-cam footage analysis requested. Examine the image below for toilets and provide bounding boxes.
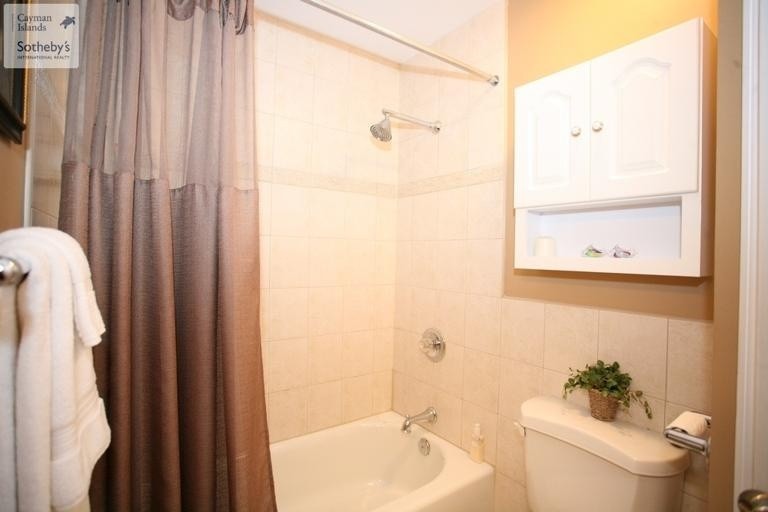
[519,396,690,512]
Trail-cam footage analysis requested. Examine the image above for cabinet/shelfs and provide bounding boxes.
[513,16,714,283]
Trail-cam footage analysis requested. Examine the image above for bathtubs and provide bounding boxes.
[236,410,498,512]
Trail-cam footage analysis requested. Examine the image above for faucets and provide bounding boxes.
[400,408,438,433]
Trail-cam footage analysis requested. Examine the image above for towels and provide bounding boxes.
[0,226,112,510]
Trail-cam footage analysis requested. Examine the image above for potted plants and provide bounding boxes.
[560,360,655,424]
